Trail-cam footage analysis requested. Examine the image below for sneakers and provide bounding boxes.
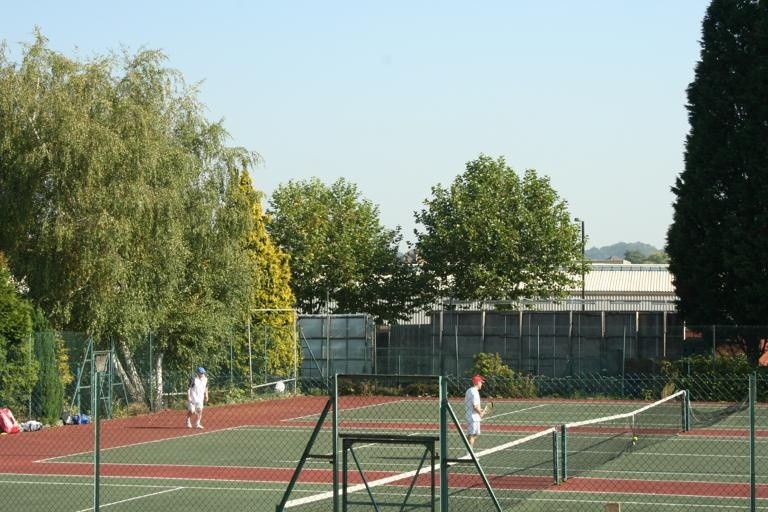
[185,417,191,428]
[195,424,204,429]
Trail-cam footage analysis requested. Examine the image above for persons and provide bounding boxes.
[464,375,485,461]
[187,367,208,429]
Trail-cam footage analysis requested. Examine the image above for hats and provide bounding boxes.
[198,367,205,373]
[472,377,485,383]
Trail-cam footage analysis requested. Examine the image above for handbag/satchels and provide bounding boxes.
[0,408,21,433]
[22,421,43,431]
